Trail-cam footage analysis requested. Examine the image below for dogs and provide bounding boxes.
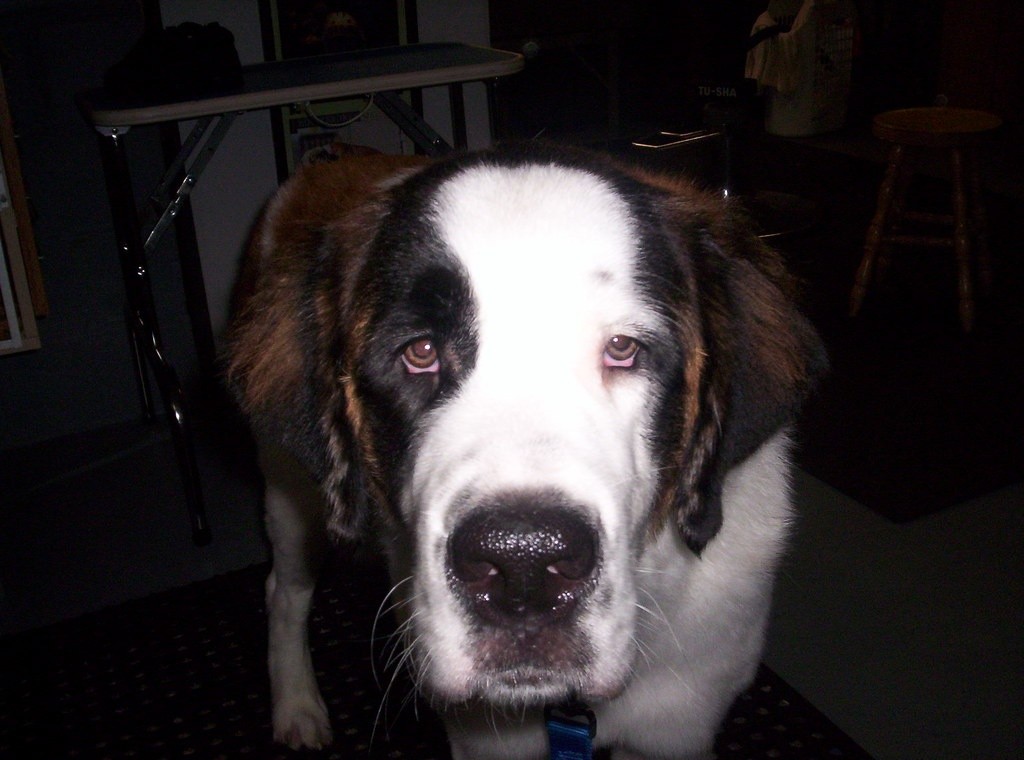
[221,141,834,759]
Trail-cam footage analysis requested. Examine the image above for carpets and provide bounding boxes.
[0,559,873,760]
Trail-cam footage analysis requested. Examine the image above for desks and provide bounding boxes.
[76,41,524,548]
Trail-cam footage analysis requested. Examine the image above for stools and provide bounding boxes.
[849,108,1003,334]
